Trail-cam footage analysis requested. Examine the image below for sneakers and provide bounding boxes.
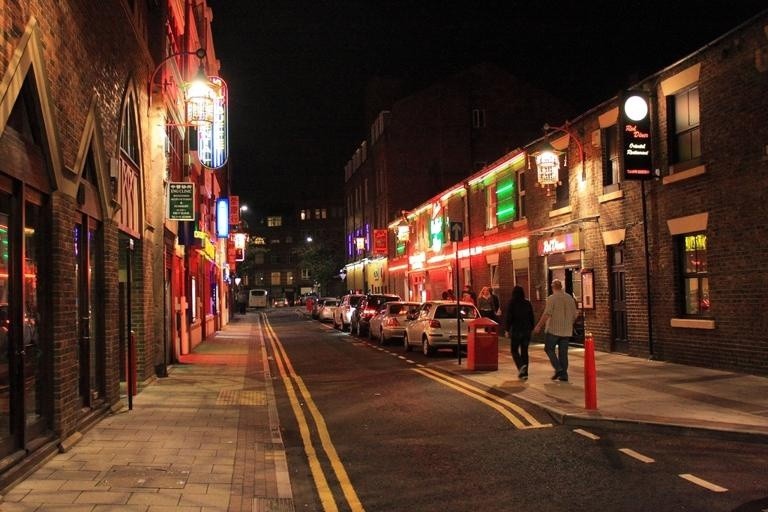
[517,364,527,377]
[559,378,568,381]
[552,373,562,380]
[520,374,528,380]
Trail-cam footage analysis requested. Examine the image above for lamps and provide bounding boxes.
[526,122,585,190]
[149,49,223,127]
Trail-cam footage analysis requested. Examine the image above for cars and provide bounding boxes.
[294,293,485,356]
[274,297,288,308]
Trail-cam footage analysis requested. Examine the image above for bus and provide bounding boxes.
[249,289,269,308]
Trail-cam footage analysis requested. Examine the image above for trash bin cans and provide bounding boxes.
[467,317,499,371]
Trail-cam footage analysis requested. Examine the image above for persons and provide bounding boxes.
[441,284,500,332]
[238,290,248,315]
[534,278,578,381]
[504,285,535,380]
